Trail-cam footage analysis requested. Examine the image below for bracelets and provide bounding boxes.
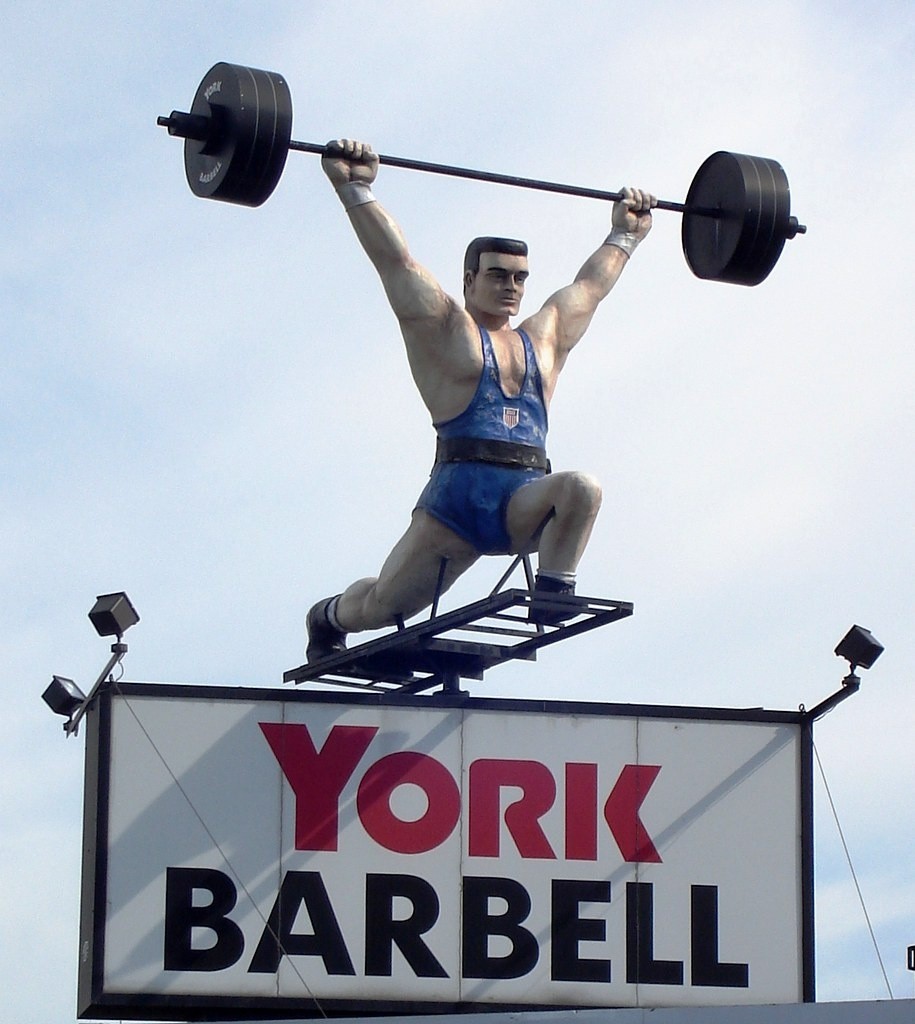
[604,223,640,261]
[335,179,375,210]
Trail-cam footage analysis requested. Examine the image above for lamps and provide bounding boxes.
[44,675,87,737]
[66,590,140,732]
[804,624,885,723]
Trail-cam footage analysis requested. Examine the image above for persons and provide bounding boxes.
[305,135,657,672]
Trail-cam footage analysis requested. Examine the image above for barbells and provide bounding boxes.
[158,59,806,290]
